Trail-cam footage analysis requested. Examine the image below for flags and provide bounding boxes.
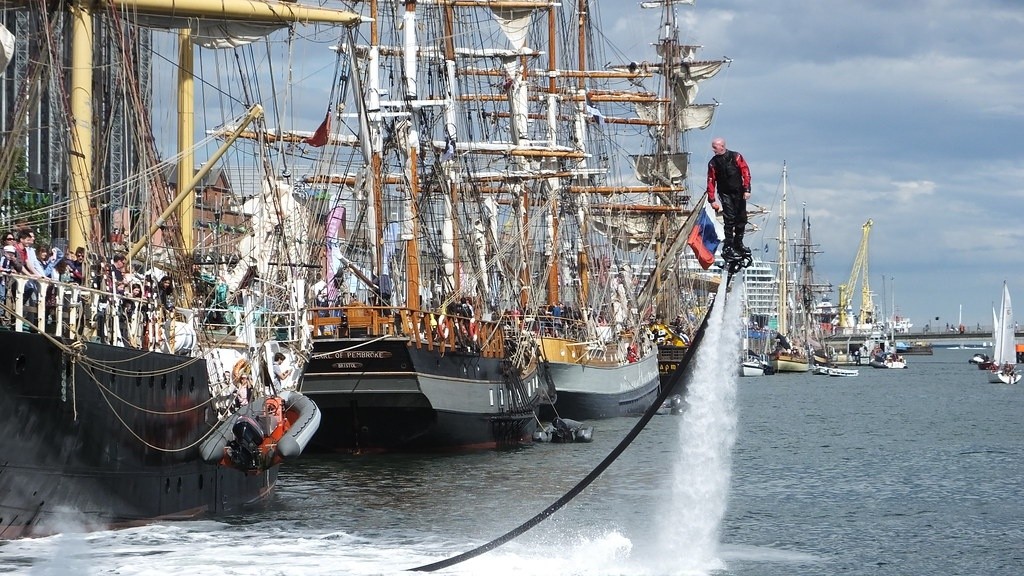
[687,192,725,270]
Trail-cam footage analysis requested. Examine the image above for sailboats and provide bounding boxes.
[0,1,1024,547]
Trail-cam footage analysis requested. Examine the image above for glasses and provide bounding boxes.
[77,255,84,258]
[121,261,126,265]
[6,238,14,240]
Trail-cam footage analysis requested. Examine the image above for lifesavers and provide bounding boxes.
[157,322,197,354]
[469,317,478,342]
[231,358,251,390]
[437,314,449,339]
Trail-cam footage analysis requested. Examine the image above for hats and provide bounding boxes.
[3,245,16,253]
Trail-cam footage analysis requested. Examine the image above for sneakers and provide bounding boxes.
[721,246,742,259]
[734,241,751,255]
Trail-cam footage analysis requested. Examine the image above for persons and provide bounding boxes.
[707,137,751,263]
[273,353,293,394]
[0,227,175,350]
[523,302,584,341]
[460,297,474,318]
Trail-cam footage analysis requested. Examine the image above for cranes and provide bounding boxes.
[839,217,877,335]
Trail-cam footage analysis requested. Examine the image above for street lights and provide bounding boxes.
[890,275,897,324]
